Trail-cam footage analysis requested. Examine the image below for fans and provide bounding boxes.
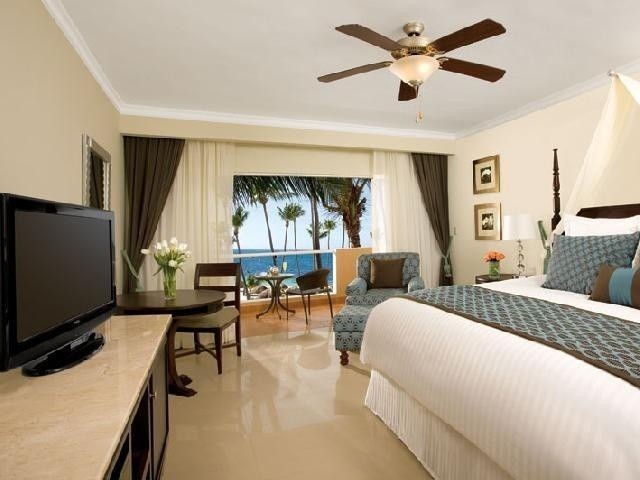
[317,17,509,102]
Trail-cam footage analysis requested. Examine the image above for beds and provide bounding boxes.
[359,148,639,480]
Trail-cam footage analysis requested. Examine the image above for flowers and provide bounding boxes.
[483,252,506,273]
[140,235,192,294]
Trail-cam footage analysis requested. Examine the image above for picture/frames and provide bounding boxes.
[472,154,500,194]
[474,202,502,240]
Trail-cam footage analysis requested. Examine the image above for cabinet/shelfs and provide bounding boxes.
[0,312,174,479]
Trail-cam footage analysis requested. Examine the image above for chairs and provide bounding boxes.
[171,259,243,374]
[334,252,425,368]
[286,268,335,326]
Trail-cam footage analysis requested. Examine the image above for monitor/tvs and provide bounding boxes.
[0,192,116,377]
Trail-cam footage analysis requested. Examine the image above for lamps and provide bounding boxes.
[502,213,537,279]
[389,52,442,95]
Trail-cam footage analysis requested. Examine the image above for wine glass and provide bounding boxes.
[283,262,287,275]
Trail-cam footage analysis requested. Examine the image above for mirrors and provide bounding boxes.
[81,133,112,211]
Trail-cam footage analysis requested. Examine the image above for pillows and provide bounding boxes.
[591,266,639,310]
[540,232,639,296]
[562,213,639,268]
[368,258,406,289]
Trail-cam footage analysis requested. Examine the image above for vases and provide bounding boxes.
[488,262,500,277]
[163,275,176,301]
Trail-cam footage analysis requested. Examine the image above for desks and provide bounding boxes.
[255,273,297,321]
[116,288,226,396]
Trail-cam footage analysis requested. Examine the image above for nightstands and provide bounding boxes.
[475,274,515,284]
